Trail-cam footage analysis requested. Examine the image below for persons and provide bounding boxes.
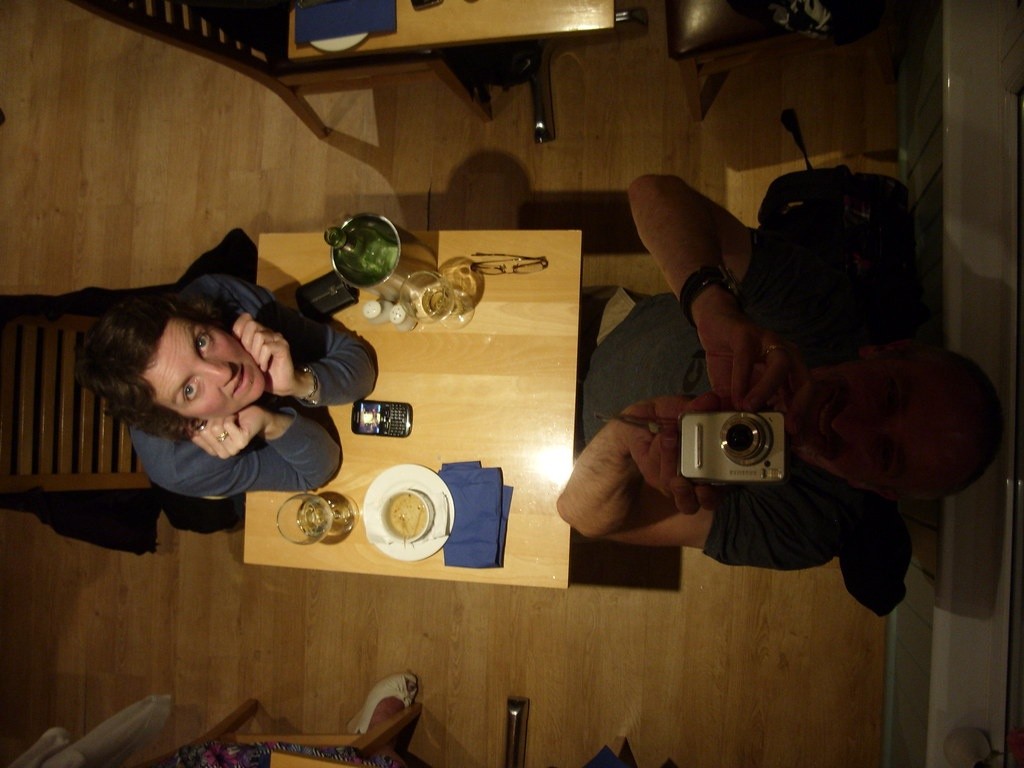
[557,175,1005,571]
[157,673,418,767]
[86,276,375,496]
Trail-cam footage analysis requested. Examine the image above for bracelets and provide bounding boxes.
[299,366,318,400]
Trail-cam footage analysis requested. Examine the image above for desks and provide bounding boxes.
[289,0,647,143]
[241,227,583,589]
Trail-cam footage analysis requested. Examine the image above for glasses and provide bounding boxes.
[470,253,549,275]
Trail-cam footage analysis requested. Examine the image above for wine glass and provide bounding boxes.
[277,490,360,545]
[399,270,474,331]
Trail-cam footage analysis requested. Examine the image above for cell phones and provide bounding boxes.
[351,400,412,438]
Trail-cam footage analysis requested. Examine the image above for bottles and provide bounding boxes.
[364,301,396,324]
[390,305,417,331]
[323,225,398,280]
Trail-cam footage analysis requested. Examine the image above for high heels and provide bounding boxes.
[346,673,417,734]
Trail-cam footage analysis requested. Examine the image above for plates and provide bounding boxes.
[363,464,454,562]
[310,33,370,52]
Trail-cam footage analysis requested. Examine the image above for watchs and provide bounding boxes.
[679,264,741,329]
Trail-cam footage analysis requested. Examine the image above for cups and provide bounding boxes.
[330,213,437,301]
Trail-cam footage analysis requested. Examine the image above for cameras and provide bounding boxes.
[677,410,785,486]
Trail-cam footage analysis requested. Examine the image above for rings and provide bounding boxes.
[217,432,227,441]
[766,344,784,354]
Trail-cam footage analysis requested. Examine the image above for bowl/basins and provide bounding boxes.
[380,491,434,544]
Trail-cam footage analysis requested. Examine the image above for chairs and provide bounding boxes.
[665,0,895,122]
[0,315,153,494]
[128,698,421,768]
[70,0,492,140]
